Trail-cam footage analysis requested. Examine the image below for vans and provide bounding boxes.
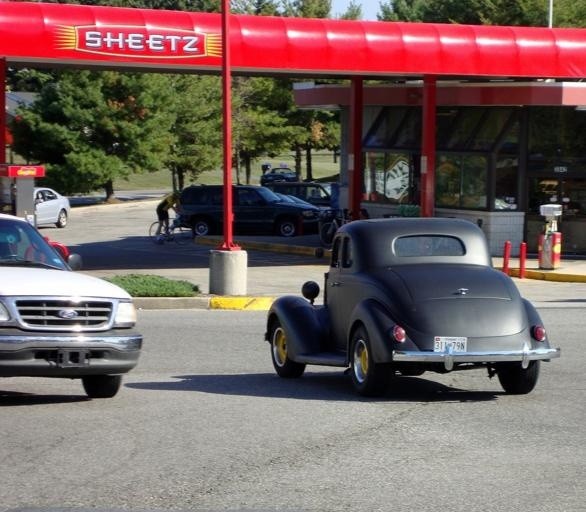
[171,167,337,238]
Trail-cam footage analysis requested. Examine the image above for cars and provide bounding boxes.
[25,188,71,228]
[0,213,144,398]
[262,215,562,397]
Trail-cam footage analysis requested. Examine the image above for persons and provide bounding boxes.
[153,189,185,243]
[359,209,369,220]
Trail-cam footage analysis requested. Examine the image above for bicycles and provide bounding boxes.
[319,207,353,247]
[149,209,194,246]
